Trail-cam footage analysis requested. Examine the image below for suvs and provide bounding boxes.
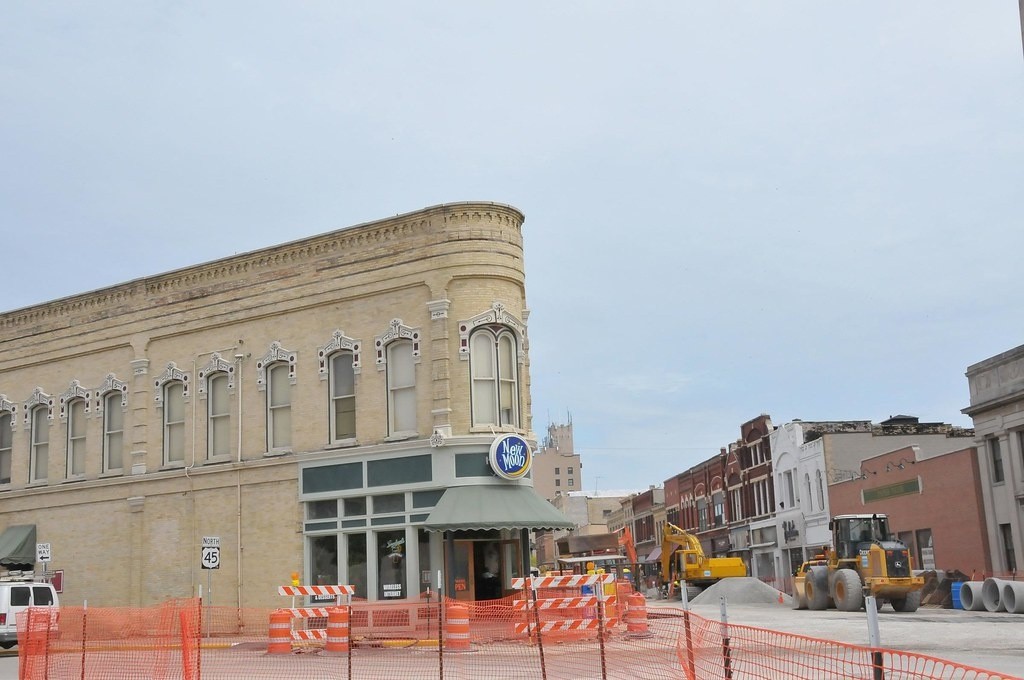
[0,570,62,647]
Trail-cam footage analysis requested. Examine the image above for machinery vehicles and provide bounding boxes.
[792,513,925,612]
[659,520,747,601]
[551,525,640,590]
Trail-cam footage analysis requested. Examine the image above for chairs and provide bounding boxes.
[860,529,872,542]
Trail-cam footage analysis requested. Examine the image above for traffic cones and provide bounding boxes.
[779,593,783,603]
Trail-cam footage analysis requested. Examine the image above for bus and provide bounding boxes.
[530,567,540,577]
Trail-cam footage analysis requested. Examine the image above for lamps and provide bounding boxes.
[851,472,866,482]
[899,458,915,469]
[862,469,877,479]
[886,461,900,472]
